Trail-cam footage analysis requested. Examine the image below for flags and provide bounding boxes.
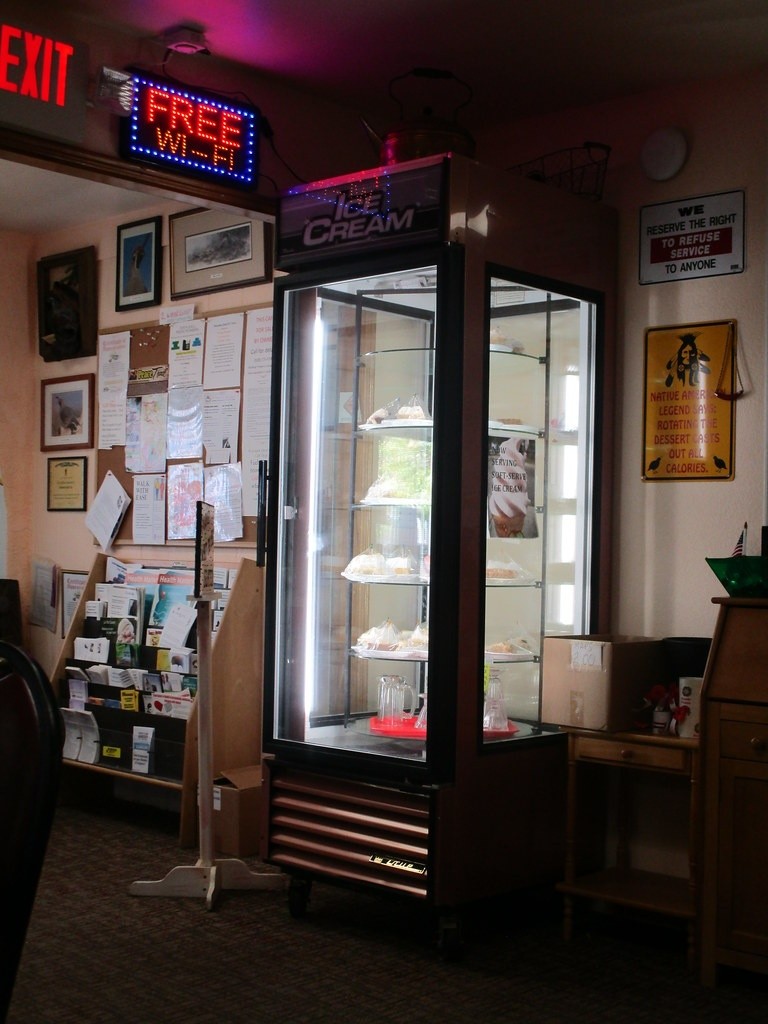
[725,530,752,583]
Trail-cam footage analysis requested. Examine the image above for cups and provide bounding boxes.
[379,676,417,727]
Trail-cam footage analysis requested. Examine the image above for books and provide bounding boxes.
[58,557,238,775]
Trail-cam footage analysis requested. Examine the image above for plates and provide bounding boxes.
[486,578,536,585]
[360,499,432,506]
[490,344,513,352]
[485,644,533,661]
[341,572,430,585]
[489,422,539,439]
[351,646,428,660]
[358,420,434,429]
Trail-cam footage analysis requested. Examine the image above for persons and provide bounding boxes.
[56,396,64,408]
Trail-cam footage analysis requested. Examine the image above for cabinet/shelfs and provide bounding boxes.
[255,151,624,966]
[556,723,708,977]
[691,597,768,990]
[42,551,265,845]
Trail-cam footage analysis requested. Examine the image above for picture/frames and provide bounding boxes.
[46,455,87,512]
[113,214,162,312]
[166,206,273,300]
[40,372,95,452]
[34,244,99,363]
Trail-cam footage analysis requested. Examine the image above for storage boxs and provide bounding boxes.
[212,765,261,857]
[538,632,701,733]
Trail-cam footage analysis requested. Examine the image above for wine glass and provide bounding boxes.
[416,694,427,728]
[483,668,509,731]
